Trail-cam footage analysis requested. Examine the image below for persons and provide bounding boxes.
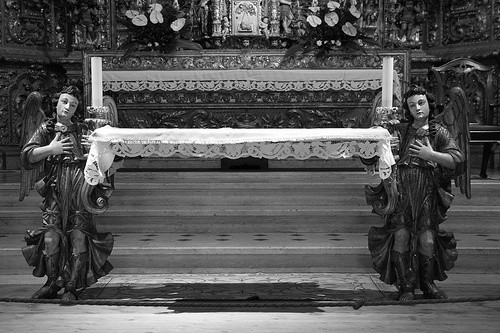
[74,1,99,45]
[260,15,270,41]
[222,15,232,42]
[364,85,471,303]
[394,0,422,43]
[18,85,114,305]
[205,37,287,48]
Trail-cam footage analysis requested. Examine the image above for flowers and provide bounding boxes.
[280,0,380,62]
[114,0,203,61]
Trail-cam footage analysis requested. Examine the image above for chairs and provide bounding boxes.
[428,56,500,199]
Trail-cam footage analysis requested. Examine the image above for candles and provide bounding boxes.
[381,55,395,108]
[91,53,103,107]
